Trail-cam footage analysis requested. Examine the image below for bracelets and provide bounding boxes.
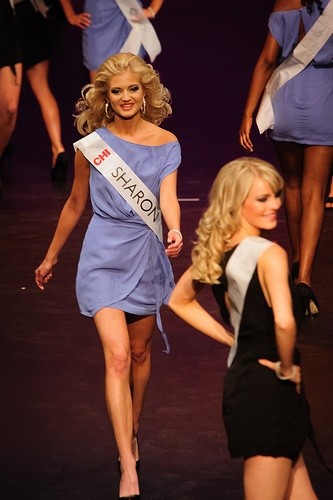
[169,229,182,236]
[276,361,297,380]
[147,6,156,19]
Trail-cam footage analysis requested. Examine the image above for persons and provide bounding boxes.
[35,53,183,500]
[0,0,66,185]
[168,157,319,500]
[239,0,333,319]
[61,0,163,85]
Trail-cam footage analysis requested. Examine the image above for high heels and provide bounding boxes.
[292,262,300,278]
[118,436,140,500]
[296,282,319,320]
[51,152,68,188]
